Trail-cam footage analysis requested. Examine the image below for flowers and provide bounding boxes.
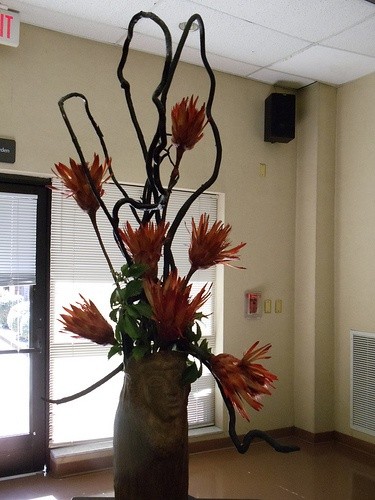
[43,11,275,441]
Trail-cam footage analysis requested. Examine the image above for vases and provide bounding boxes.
[109,349,199,499]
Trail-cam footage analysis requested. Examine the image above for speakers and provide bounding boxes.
[264,92,295,144]
